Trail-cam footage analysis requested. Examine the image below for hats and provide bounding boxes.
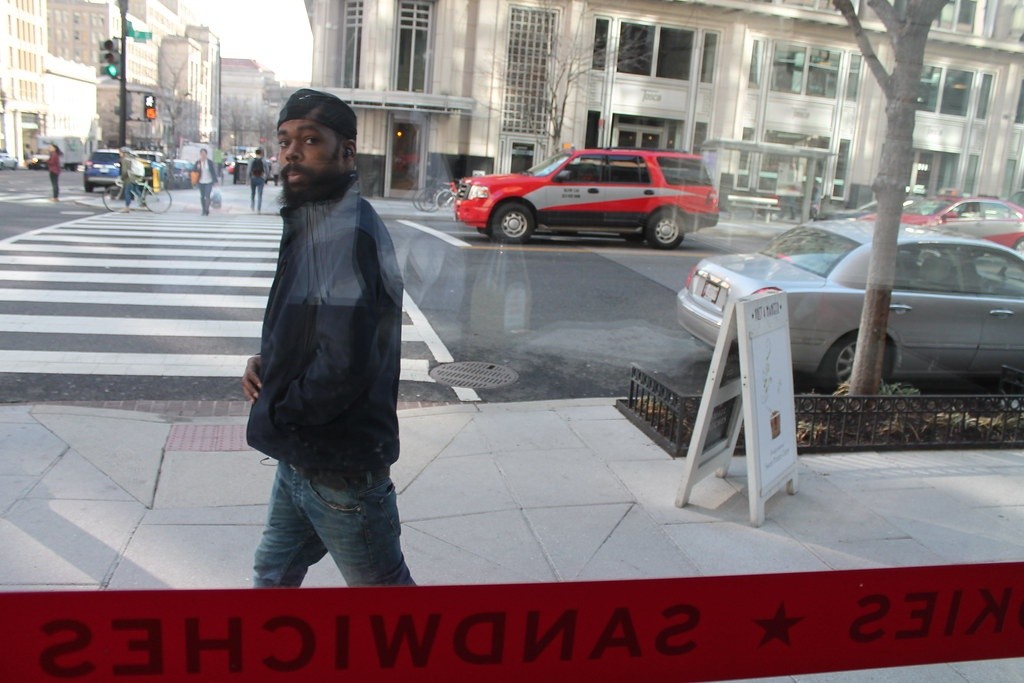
[276,89,359,140]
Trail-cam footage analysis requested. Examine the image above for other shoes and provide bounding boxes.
[120,209,129,213]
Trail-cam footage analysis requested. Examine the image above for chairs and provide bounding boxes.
[897,247,977,286]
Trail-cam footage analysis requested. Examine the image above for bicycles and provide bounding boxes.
[413,179,458,214]
[102,175,173,215]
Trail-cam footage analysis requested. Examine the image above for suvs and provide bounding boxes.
[452,146,721,250]
[133,150,166,190]
[83,148,123,193]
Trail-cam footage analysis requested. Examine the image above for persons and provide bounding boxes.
[774,197,798,224]
[47,144,62,203]
[809,181,821,220]
[233,157,240,184]
[24,143,35,169]
[118,146,147,214]
[242,89,417,588]
[195,149,216,215]
[271,158,280,187]
[246,150,268,214]
[871,172,882,203]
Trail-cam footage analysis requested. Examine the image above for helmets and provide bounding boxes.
[119,147,132,154]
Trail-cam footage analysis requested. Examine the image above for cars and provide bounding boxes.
[0,151,18,171]
[673,217,1024,414]
[809,187,1024,265]
[180,141,270,184]
[165,158,195,190]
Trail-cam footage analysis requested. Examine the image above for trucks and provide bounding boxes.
[28,136,85,172]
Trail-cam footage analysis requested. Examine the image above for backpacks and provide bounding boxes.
[125,156,145,183]
[252,156,264,177]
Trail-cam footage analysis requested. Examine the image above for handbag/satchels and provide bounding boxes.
[211,191,222,209]
[190,161,201,184]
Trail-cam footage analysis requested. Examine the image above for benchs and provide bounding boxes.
[727,195,781,223]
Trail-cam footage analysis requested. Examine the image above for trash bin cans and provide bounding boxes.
[237,163,247,183]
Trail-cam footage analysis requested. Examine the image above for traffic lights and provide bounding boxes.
[98,40,108,73]
[143,96,157,121]
[105,38,120,77]
